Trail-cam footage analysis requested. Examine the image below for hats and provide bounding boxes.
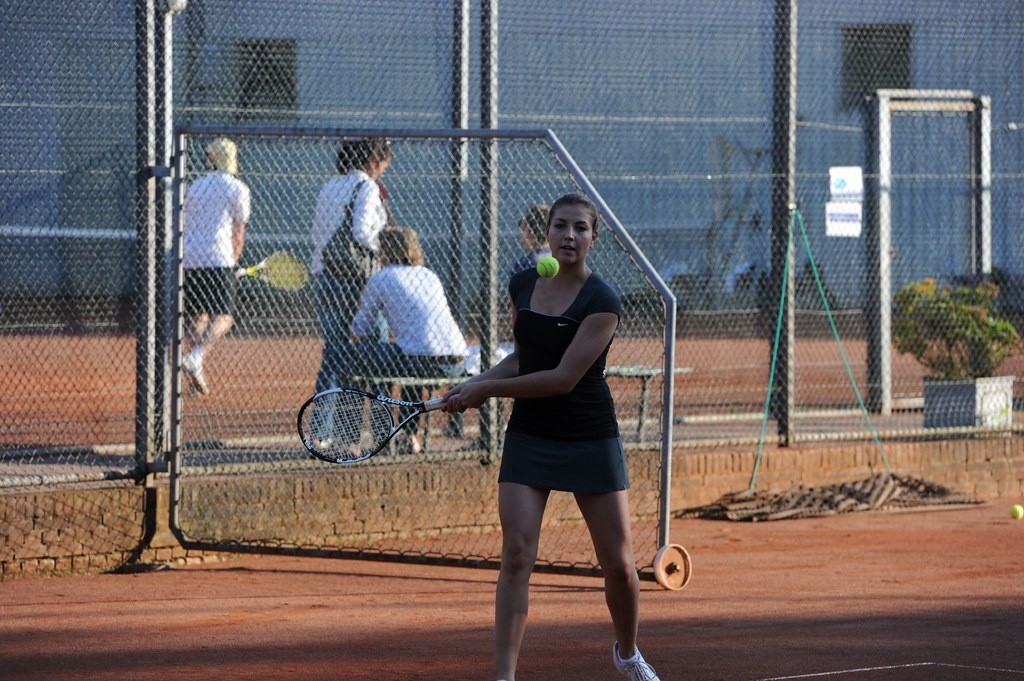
[205,137,238,175]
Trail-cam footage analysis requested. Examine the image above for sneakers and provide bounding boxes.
[612,639,659,681]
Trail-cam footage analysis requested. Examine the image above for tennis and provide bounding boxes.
[1010,505,1023,519]
[536,256,559,279]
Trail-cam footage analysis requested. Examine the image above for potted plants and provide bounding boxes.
[894,278,1018,439]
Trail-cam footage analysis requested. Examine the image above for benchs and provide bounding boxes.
[345,364,692,457]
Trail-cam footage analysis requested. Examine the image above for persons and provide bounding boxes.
[301,140,392,460]
[513,202,551,277]
[330,222,467,455]
[439,197,656,680]
[183,137,249,399]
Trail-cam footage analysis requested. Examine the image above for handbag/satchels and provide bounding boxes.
[320,179,384,292]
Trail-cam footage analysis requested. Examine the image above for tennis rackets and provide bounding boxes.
[235,251,308,292]
[296,385,462,463]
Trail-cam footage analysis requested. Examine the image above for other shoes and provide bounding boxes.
[308,409,466,465]
[181,352,209,396]
